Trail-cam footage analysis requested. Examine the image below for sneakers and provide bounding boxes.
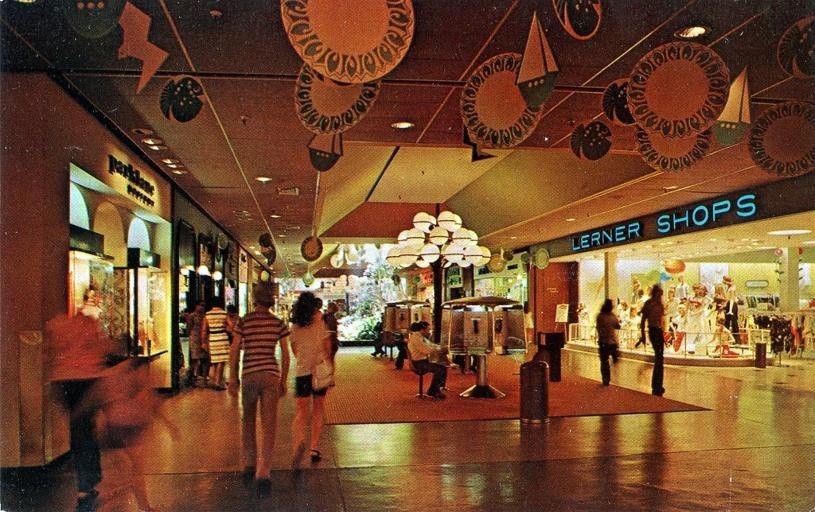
[243,466,272,501]
[427,388,445,399]
[77,490,98,512]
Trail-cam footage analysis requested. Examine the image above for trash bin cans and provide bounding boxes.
[755,343,766,368]
[520,361,551,424]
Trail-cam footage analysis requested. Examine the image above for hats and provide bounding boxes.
[723,276,732,285]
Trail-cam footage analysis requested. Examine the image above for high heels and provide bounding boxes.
[207,380,227,391]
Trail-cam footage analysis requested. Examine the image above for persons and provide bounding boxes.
[278,303,290,325]
[577,274,741,357]
[44,278,182,512]
[371,326,386,355]
[183,295,241,392]
[495,327,509,352]
[229,281,291,500]
[407,322,449,400]
[597,299,620,386]
[634,285,665,396]
[421,322,449,391]
[289,292,339,477]
[767,303,775,312]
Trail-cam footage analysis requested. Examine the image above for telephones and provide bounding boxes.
[400,314,404,321]
[415,313,418,321]
[473,320,478,334]
[496,318,503,333]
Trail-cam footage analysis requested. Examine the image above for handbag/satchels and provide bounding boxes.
[312,362,335,389]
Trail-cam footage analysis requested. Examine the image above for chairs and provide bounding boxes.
[403,346,431,399]
[382,331,399,360]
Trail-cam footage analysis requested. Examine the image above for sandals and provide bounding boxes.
[309,449,322,462]
